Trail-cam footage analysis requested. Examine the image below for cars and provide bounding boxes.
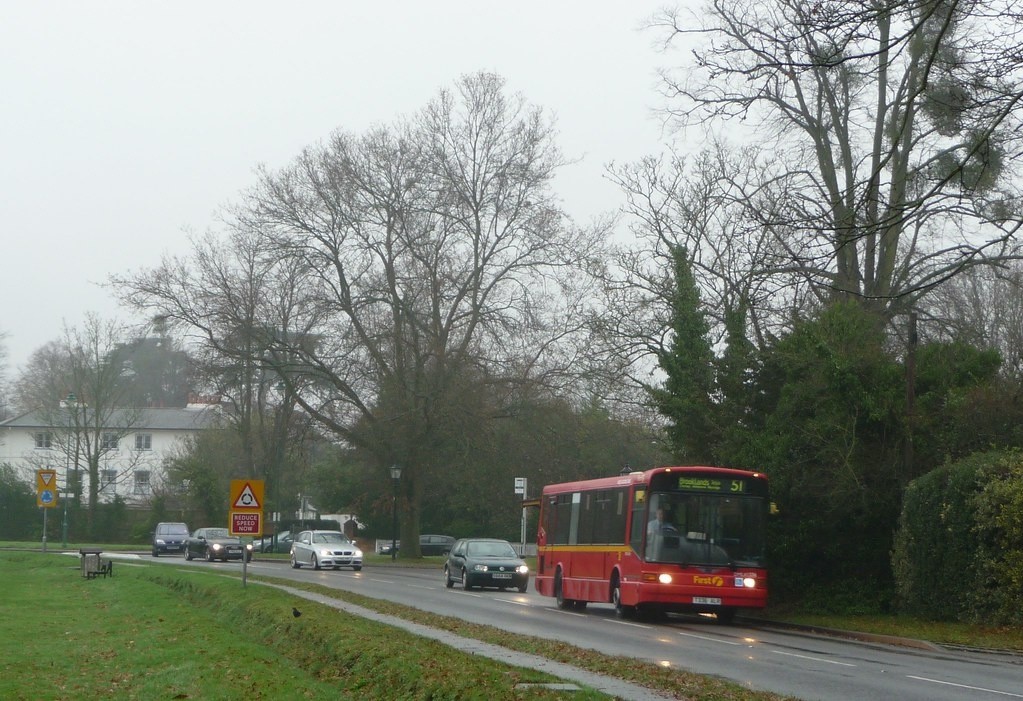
[181,526,254,562]
[289,530,364,571]
[379,534,457,556]
[257,530,293,554]
[443,538,530,593]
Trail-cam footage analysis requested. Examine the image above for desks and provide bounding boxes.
[86,571,108,580]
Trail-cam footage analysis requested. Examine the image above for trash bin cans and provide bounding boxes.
[79,547,103,577]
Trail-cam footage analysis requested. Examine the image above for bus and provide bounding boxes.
[533,465,780,624]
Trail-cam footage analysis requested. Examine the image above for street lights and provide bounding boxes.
[389,463,402,559]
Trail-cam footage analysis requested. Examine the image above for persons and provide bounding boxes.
[646,508,678,536]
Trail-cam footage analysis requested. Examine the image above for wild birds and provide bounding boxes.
[291,606,302,618]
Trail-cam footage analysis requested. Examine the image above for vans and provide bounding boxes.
[149,521,191,558]
